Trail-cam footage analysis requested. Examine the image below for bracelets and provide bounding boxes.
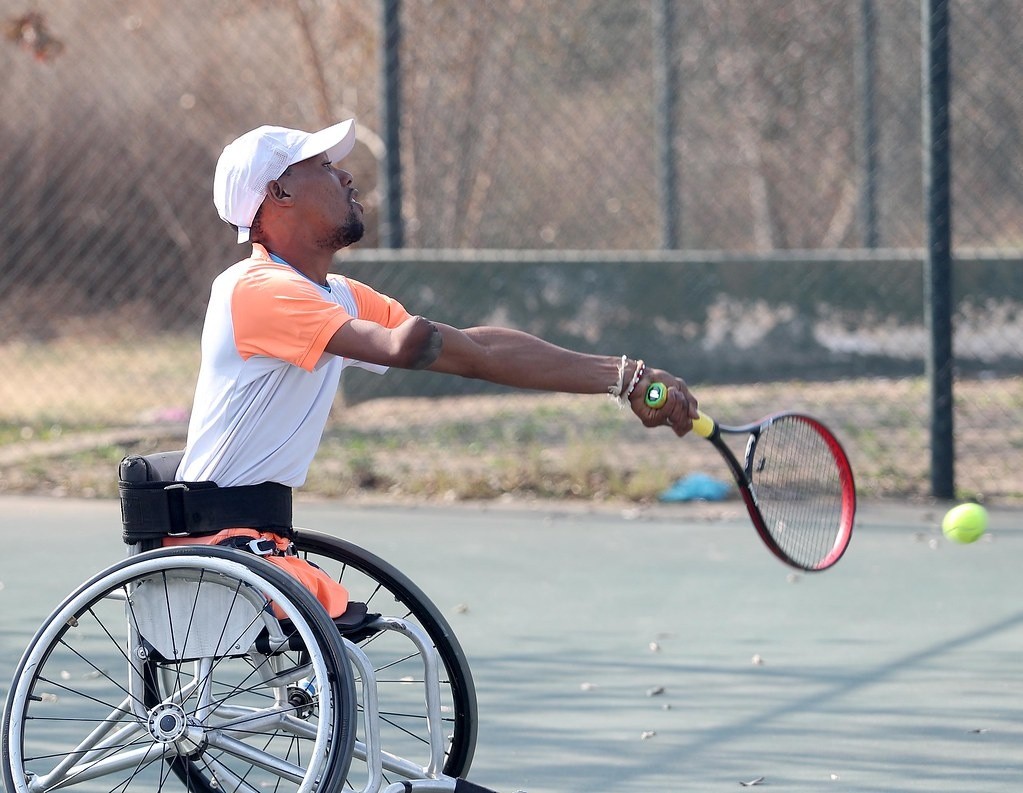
[612,355,645,407]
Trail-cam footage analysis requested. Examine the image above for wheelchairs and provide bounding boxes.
[1,448,498,793]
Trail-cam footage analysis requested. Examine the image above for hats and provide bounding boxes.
[212,117,356,245]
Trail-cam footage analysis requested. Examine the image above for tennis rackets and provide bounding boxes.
[644,382,857,573]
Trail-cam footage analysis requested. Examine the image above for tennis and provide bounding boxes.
[943,503,991,544]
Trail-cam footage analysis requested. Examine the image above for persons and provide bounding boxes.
[163,118,698,623]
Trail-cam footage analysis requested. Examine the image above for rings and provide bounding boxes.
[667,417,673,426]
